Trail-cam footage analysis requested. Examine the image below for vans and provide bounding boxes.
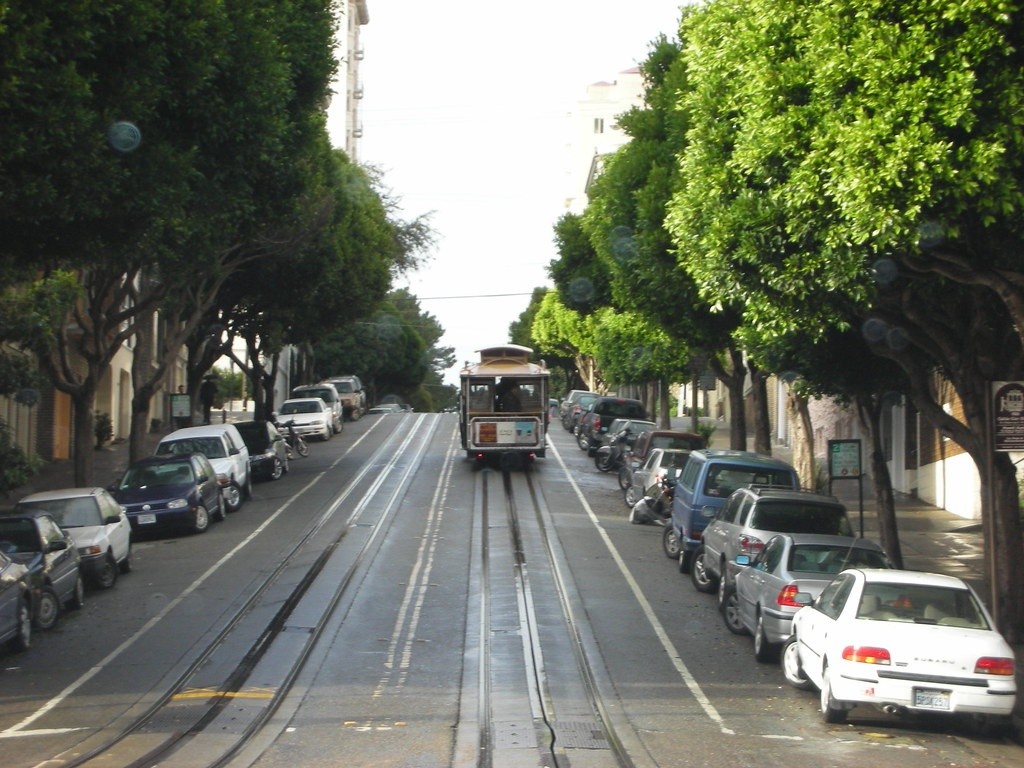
[662,448,802,571]
[152,424,253,503]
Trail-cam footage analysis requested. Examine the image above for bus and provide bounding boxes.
[456,342,551,466]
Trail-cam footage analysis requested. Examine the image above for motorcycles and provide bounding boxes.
[644,465,681,520]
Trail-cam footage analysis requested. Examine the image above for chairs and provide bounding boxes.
[924,603,956,621]
[176,467,190,479]
[793,554,807,570]
[858,594,882,615]
[141,471,158,487]
[800,561,820,571]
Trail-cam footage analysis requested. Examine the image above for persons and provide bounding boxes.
[201,379,218,424]
[176,385,188,429]
[476,378,521,412]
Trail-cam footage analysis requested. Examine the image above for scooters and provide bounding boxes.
[271,420,310,459]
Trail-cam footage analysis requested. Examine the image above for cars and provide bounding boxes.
[369,404,404,414]
[317,372,368,420]
[779,568,1017,735]
[227,419,288,480]
[0,511,85,631]
[561,389,705,493]
[15,486,133,592]
[624,447,691,506]
[725,533,898,661]
[399,402,415,413]
[274,397,335,440]
[107,451,226,538]
[0,548,47,653]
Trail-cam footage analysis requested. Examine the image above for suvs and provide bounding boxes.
[291,383,345,433]
[689,485,859,611]
